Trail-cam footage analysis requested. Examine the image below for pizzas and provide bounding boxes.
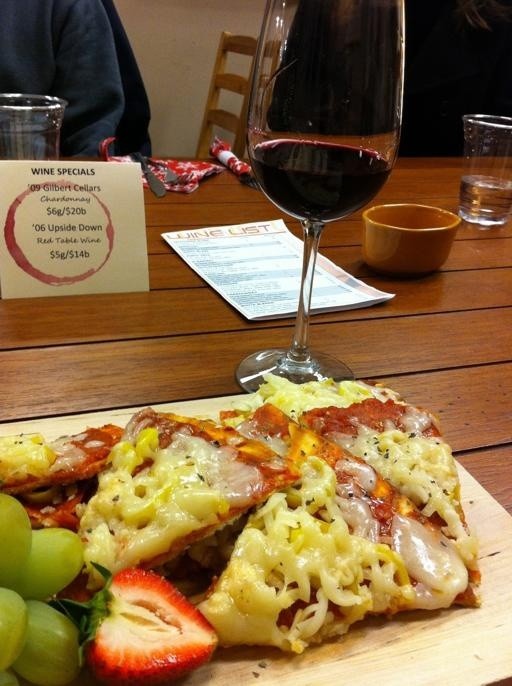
[0,372,482,652]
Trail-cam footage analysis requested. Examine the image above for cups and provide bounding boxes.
[235,1,406,390]
[0,93,67,158]
[462,114,510,225]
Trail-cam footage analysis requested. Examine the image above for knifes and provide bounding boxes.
[132,153,165,198]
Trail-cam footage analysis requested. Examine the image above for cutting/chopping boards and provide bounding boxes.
[0,385,512,686]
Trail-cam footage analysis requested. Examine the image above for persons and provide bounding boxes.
[268,0,511,159]
[1,0,128,160]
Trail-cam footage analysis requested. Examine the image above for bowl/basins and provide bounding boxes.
[362,204,460,276]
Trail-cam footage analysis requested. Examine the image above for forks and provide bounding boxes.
[155,162,195,186]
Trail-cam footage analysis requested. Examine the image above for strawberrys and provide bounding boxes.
[49,561,219,686]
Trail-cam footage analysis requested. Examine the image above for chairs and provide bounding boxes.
[194,30,283,161]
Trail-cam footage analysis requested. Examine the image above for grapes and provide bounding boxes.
[0,492,84,686]
